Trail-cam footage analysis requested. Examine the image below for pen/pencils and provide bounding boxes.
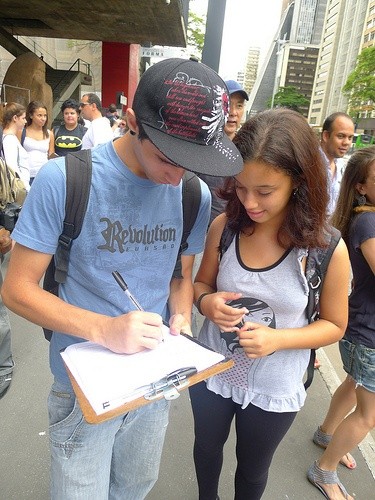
[112,270,165,342]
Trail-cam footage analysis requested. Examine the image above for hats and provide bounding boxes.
[132,58,243,176]
[225,80,249,101]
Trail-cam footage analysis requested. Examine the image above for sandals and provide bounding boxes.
[312,427,356,469]
[306,460,355,500]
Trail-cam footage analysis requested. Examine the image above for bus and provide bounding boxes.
[350,132,375,153]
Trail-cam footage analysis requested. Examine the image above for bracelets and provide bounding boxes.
[196,291,216,316]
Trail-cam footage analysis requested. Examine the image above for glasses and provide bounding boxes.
[80,103,92,107]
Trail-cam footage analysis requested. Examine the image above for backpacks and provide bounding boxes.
[0,153,28,231]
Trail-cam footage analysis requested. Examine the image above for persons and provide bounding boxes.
[0,58,246,500]
[0,93,130,230]
[189,81,249,284]
[187,106,353,500]
[313,112,355,371]
[306,146,375,500]
[0,226,16,398]
